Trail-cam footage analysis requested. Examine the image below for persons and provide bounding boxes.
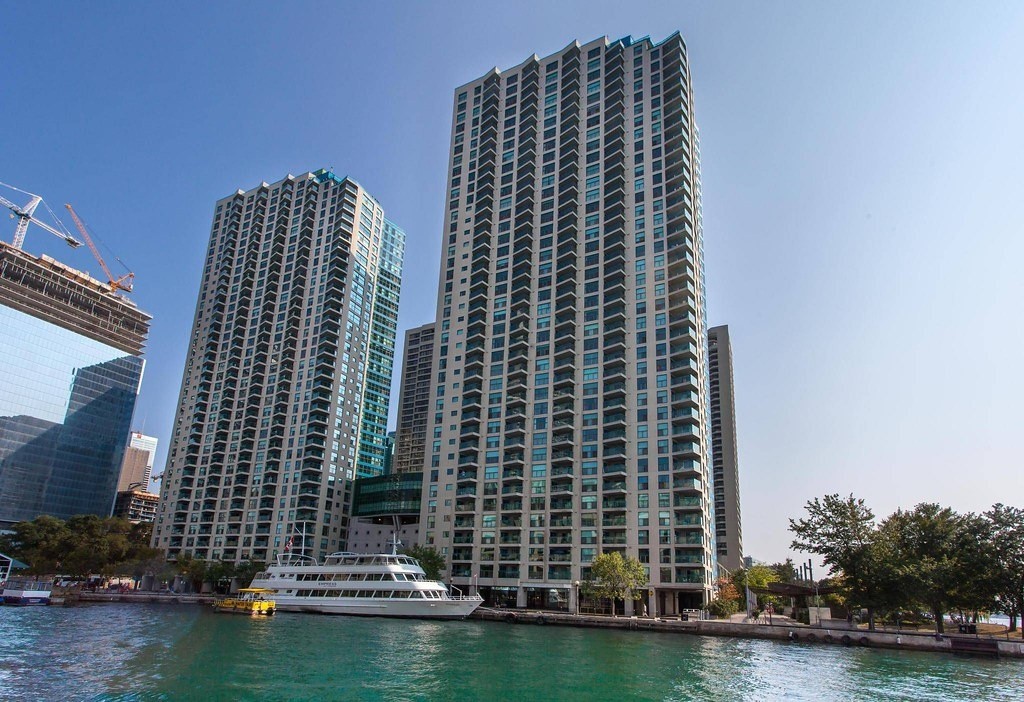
[642,602,649,618]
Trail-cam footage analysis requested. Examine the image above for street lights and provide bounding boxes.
[575,580,579,616]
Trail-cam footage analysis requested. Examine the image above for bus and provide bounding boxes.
[52,575,104,590]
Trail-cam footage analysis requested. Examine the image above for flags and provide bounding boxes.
[284,536,294,552]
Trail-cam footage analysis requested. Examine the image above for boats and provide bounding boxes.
[0,588,52,607]
[242,521,485,620]
[212,588,277,615]
